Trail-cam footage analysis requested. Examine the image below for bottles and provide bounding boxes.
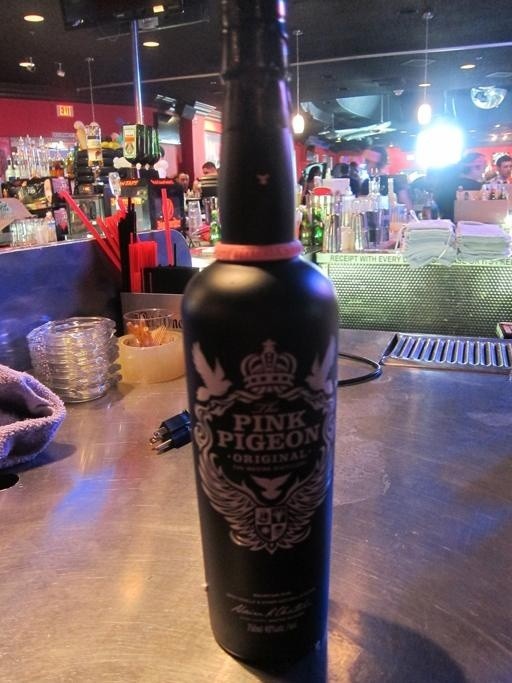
[4,131,74,182]
[41,210,58,243]
[121,123,161,169]
[298,168,441,255]
[208,197,222,246]
[456,184,506,200]
[180,0,342,668]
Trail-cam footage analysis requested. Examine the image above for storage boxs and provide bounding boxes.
[454,190,509,223]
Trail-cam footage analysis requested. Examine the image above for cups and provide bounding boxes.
[123,307,177,346]
[9,217,46,248]
[186,200,203,242]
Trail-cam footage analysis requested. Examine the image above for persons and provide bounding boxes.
[306,144,512,248]
[19,179,28,186]
[193,161,220,194]
[176,168,193,211]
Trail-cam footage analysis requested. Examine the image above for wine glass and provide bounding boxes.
[85,125,105,186]
